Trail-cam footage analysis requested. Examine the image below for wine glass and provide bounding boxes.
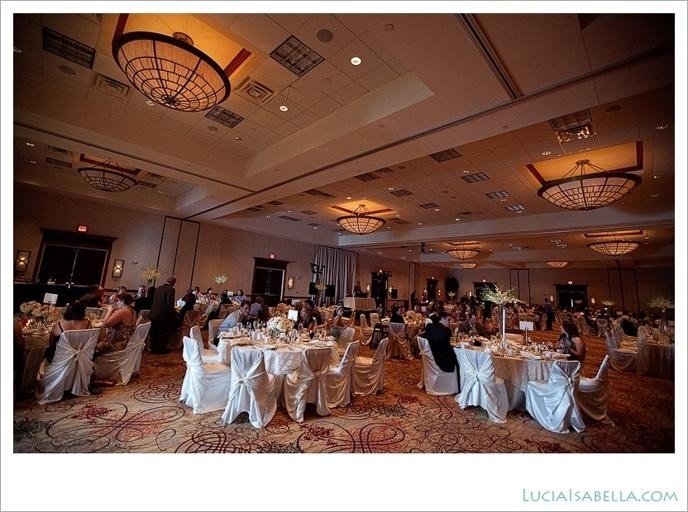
[22,312,103,335]
[449,333,566,360]
[426,297,534,333]
[588,311,673,344]
[191,293,425,351]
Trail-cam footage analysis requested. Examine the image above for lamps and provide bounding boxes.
[587,230,641,256]
[458,263,476,269]
[112,259,125,279]
[447,245,480,260]
[77,160,138,193]
[337,204,385,235]
[544,261,569,269]
[109,32,231,113]
[538,159,642,211]
[16,250,31,272]
[288,277,294,289]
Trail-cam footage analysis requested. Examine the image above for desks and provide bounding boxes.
[343,297,375,312]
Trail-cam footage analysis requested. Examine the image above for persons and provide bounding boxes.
[16,276,675,396]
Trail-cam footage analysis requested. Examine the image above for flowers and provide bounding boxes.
[648,296,674,311]
[480,280,527,306]
[137,265,160,280]
